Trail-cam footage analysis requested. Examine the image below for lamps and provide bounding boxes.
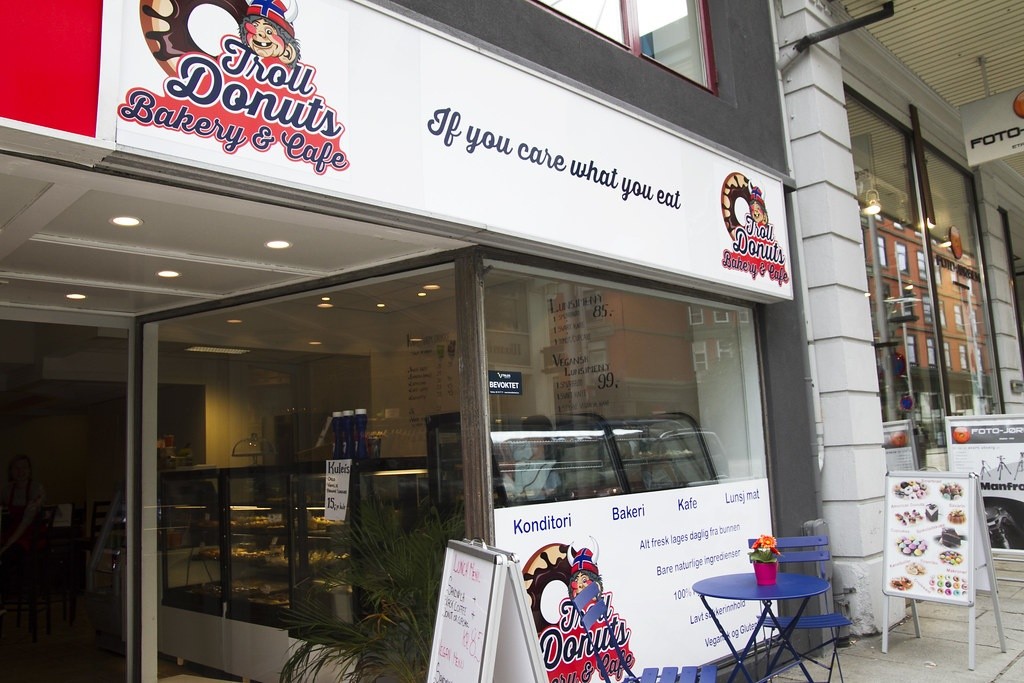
[859,175,882,216]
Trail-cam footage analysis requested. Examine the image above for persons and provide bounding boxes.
[0,453,49,616]
[501,415,564,503]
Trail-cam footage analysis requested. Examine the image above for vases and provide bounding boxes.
[753,562,777,586]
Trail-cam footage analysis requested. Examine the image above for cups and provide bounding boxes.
[164,435,175,447]
[367,439,381,458]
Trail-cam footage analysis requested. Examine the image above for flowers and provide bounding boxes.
[747,534,781,564]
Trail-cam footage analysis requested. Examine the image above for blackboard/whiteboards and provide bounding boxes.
[424,537,550,683]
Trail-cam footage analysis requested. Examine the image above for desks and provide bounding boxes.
[692,572,831,683]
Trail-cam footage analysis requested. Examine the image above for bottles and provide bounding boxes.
[355,409,368,459]
[332,412,345,460]
[343,410,357,459]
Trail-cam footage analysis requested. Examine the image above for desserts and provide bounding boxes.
[891,480,969,597]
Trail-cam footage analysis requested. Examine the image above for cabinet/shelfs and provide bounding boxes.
[153,409,720,683]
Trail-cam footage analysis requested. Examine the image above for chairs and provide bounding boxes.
[748,535,853,683]
[0,497,128,644]
